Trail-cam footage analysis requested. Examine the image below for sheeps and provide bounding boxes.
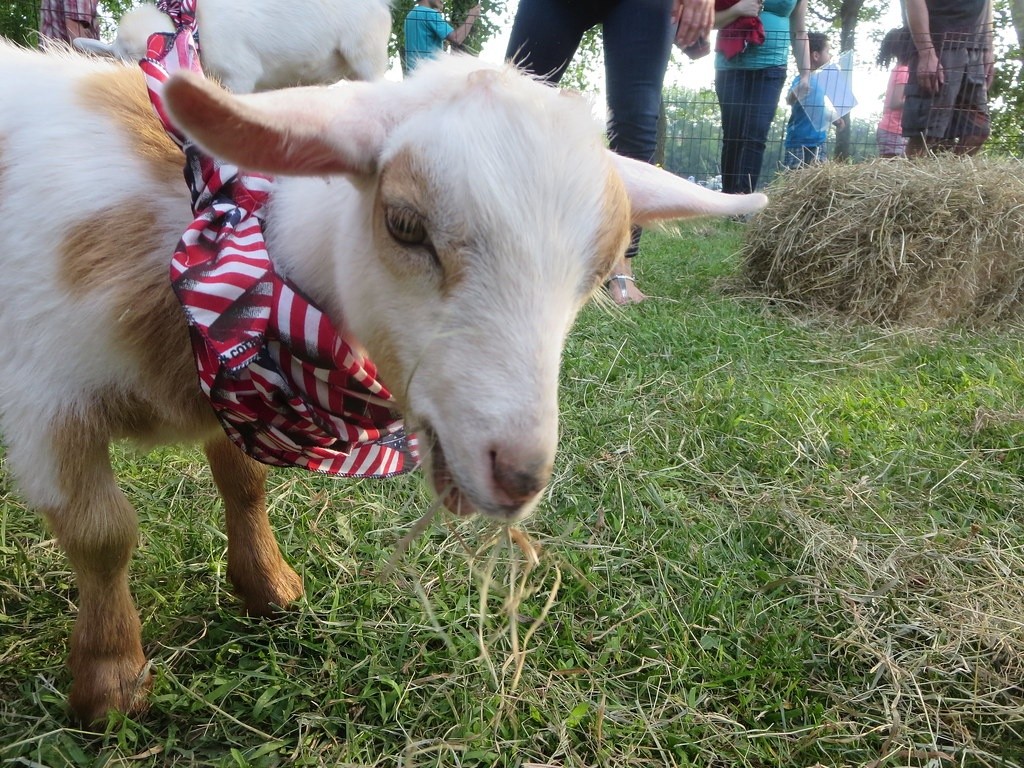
[3,2,771,730]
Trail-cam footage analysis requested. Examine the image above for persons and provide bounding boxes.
[404,0,481,75]
[41,1,100,55]
[508,1,713,306]
[714,0,811,194]
[901,0,996,161]
[876,26,914,157]
[785,31,845,168]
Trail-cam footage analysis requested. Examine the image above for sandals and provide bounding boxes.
[604,275,647,307]
